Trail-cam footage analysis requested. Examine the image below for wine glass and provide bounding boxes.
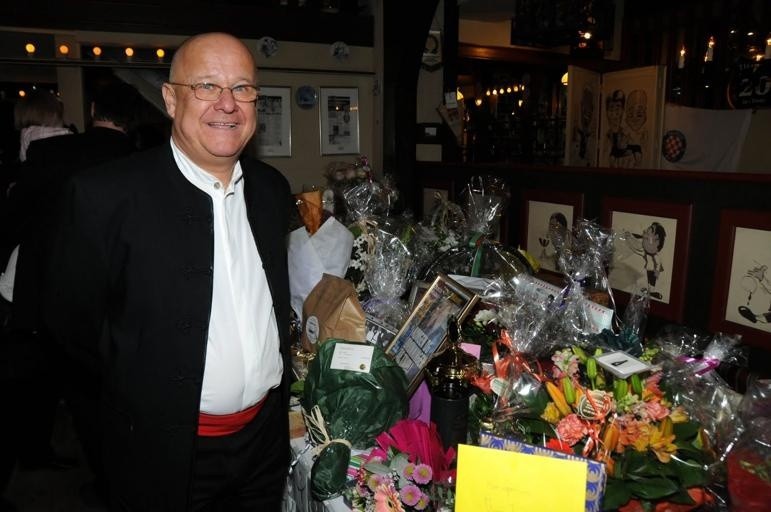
[737,258,763,294]
[535,227,551,264]
[613,220,647,242]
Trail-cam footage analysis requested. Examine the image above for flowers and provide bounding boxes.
[341,431,446,512]
[505,338,732,512]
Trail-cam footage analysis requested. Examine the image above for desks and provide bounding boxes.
[280,375,389,511]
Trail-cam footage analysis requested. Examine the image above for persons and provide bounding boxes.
[1,32,293,509]
[738,266,771,323]
[623,90,648,169]
[605,90,630,168]
[623,222,666,299]
[538,213,567,271]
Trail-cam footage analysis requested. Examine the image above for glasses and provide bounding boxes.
[169,82,260,102]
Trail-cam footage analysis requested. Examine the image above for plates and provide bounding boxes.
[295,86,318,110]
[256,36,278,60]
[330,40,351,62]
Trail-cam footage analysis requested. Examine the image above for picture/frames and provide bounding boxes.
[316,83,361,159]
[463,184,513,254]
[250,84,294,159]
[416,180,457,225]
[704,202,771,353]
[597,192,695,328]
[514,184,587,285]
